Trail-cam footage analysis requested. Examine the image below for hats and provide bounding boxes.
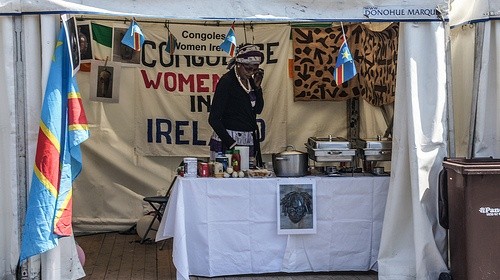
[226,43,265,70]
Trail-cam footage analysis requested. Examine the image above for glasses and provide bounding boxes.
[242,64,258,74]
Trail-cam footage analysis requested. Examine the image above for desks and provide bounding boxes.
[152,176,390,280]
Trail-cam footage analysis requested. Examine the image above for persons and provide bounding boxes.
[208,43,264,167]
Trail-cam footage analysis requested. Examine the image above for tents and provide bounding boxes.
[0,0,451,280]
[448,0,500,159]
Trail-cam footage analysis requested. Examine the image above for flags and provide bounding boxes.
[219,22,236,58]
[333,41,357,86]
[18,21,90,266]
[121,20,145,51]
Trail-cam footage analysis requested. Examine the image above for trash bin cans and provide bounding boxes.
[438,156,500,280]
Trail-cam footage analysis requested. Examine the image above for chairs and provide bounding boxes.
[141,173,179,250]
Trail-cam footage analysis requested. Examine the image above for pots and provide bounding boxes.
[272,144,309,176]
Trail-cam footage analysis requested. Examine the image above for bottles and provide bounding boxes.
[232,149,240,171]
[225,150,232,166]
[198,159,208,177]
[217,151,222,157]
[249,152,256,169]
